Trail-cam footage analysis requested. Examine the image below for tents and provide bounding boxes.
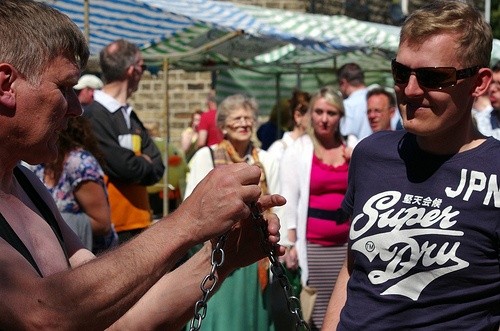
[42,0,500,218]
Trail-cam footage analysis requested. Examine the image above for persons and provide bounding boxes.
[19,40,500,331]
[322,0,500,331]
[0,0,286,331]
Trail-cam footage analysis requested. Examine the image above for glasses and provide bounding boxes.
[391,58,476,90]
[141,65,147,70]
